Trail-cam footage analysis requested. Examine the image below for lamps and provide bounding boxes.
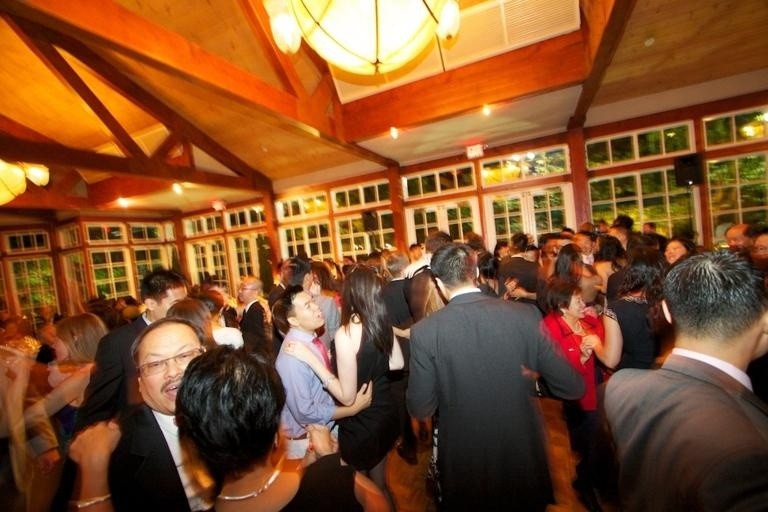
[260,0,463,75]
[0,162,51,209]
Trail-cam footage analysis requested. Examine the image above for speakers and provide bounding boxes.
[672,151,705,188]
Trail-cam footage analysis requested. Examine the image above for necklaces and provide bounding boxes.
[217,470,287,502]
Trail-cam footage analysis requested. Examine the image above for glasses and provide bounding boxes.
[134,351,199,372]
[242,286,258,292]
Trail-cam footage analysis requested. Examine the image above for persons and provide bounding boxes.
[69,341,391,512]
[1,312,109,512]
[163,296,218,351]
[466,230,510,297]
[404,241,586,512]
[498,231,540,304]
[283,264,407,512]
[598,247,767,512]
[198,286,245,351]
[50,314,213,512]
[402,230,456,326]
[321,243,426,278]
[664,236,692,264]
[587,254,668,373]
[643,221,668,253]
[238,275,270,352]
[44,266,189,511]
[541,278,605,437]
[381,250,423,464]
[271,284,340,461]
[725,222,768,270]
[278,256,341,351]
[535,213,641,277]
[309,260,342,313]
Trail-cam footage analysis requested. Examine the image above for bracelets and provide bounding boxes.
[70,493,112,511]
